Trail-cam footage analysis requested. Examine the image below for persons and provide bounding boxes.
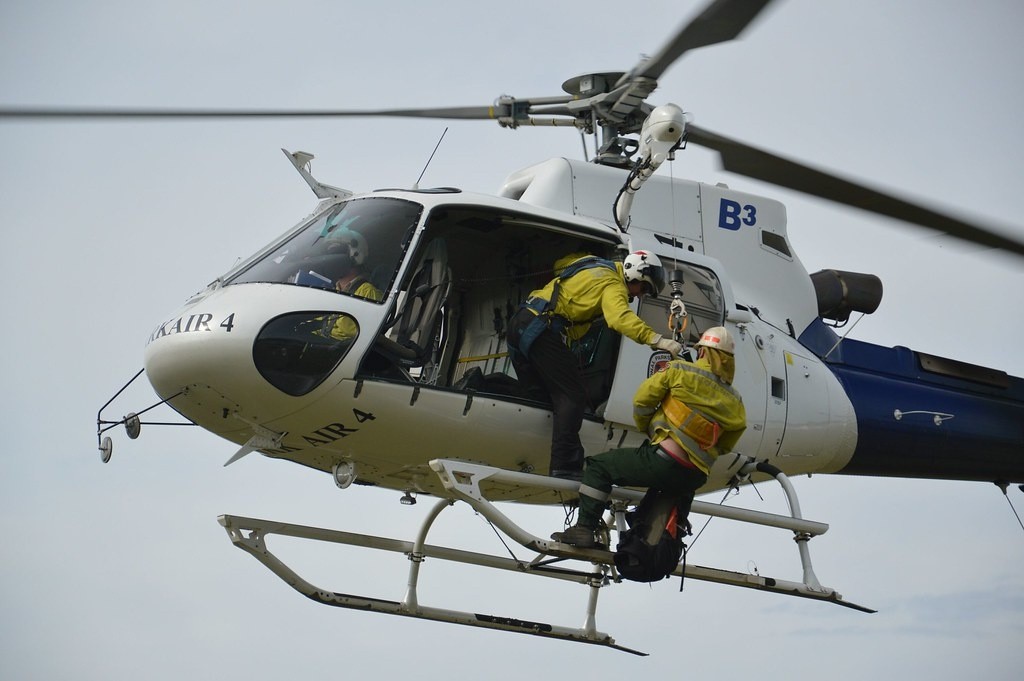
[286,228,383,395]
[454,250,682,480]
[551,326,747,547]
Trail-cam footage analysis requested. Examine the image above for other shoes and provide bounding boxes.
[549,468,586,481]
[452,366,483,391]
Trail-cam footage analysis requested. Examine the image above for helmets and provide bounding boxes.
[622,250,666,300]
[694,326,735,355]
[315,225,369,274]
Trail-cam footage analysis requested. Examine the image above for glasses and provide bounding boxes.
[635,279,650,294]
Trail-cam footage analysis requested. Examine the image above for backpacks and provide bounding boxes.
[613,476,696,582]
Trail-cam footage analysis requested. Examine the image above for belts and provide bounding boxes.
[656,448,706,485]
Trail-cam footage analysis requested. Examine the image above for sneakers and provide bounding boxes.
[550,525,594,547]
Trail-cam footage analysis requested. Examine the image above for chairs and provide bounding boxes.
[368,237,454,384]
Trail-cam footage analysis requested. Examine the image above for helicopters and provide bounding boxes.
[2,0,1024,660]
